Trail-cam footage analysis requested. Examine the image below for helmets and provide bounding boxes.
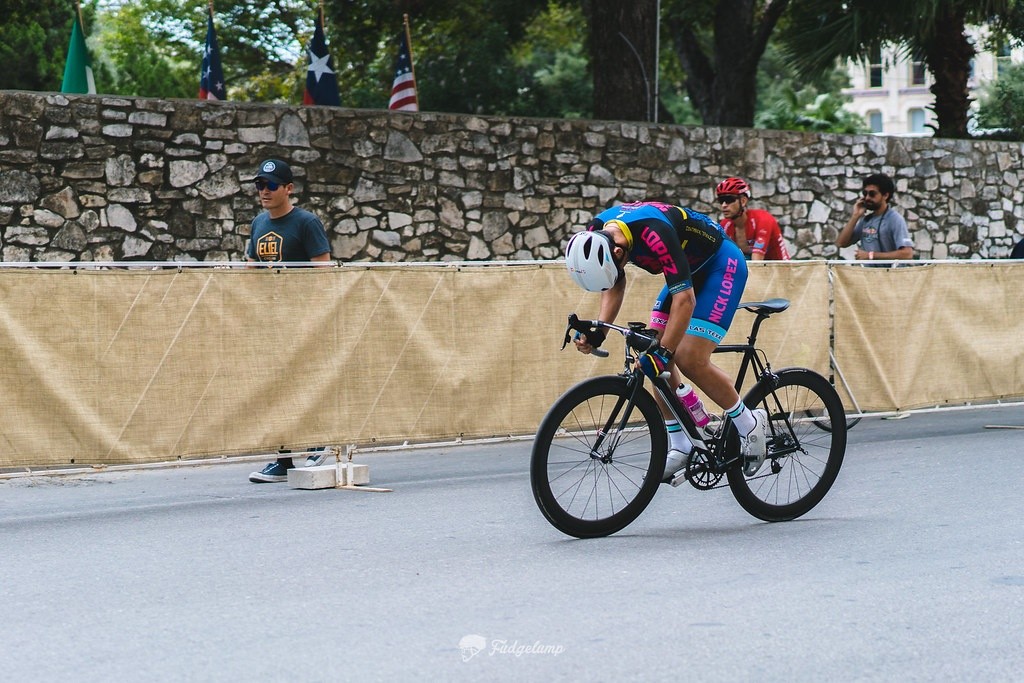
[715,177,753,199]
[564,230,621,294]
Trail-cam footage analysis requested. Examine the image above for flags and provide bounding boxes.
[304,9,340,106]
[389,23,417,111]
[200,8,226,100]
[62,21,97,95]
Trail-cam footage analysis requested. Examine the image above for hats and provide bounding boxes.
[252,158,294,185]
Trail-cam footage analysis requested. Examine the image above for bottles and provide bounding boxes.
[676,383,711,427]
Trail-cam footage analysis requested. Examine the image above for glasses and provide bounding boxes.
[716,195,742,204]
[254,181,287,191]
[862,189,881,198]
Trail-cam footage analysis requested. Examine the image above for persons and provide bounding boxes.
[564,201,767,483]
[246,159,331,482]
[716,177,790,261]
[837,173,914,420]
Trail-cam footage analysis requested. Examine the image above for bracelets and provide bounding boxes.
[869,251,873,260]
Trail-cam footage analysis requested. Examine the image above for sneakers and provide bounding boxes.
[248,462,297,483]
[303,446,331,467]
[738,408,768,477]
[641,448,702,483]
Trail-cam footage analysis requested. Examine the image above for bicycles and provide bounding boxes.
[801,376,866,431]
[529,299,847,539]
